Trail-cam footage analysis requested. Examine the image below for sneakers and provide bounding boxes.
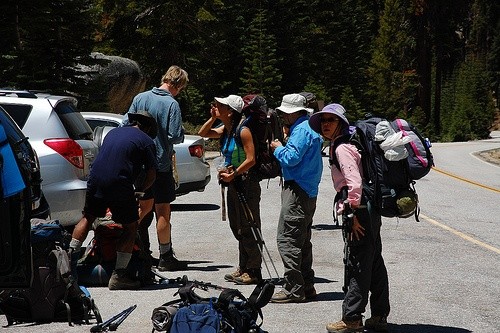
[306,286,317,300]
[157,257,188,271]
[326,319,364,333]
[363,320,388,333]
[109,270,143,290]
[224,266,246,282]
[270,289,305,303]
[234,272,263,285]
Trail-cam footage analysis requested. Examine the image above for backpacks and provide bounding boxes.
[222,94,284,181]
[151,282,275,333]
[331,117,433,218]
[29,217,95,325]
[77,221,144,287]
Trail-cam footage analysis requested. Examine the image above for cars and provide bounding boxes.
[80,111,212,198]
[0,88,99,232]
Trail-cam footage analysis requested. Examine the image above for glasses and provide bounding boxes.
[319,116,339,124]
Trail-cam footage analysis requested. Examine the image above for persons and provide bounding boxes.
[118,65,187,272]
[267,94,323,303]
[198,95,262,285]
[308,104,391,333]
[67,111,158,290]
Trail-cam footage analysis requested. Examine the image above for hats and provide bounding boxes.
[128,111,157,140]
[275,94,314,114]
[215,95,244,113]
[308,104,349,135]
